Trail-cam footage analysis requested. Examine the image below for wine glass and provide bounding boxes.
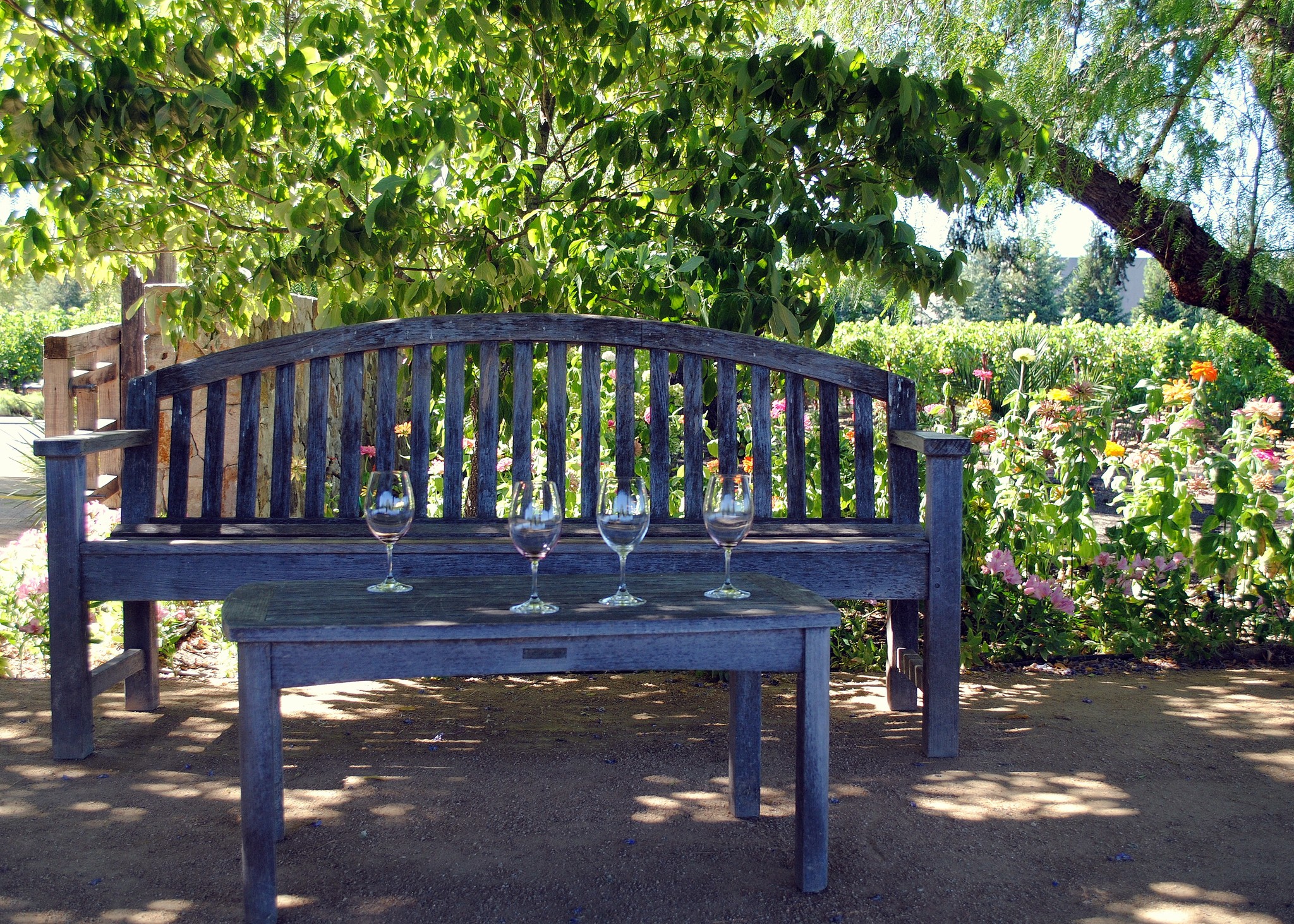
[507,480,563,614]
[702,474,754,599]
[364,471,415,593]
[595,476,651,607]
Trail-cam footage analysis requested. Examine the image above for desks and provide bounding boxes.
[219,572,847,924]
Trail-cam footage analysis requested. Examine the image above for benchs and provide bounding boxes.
[30,311,976,764]
